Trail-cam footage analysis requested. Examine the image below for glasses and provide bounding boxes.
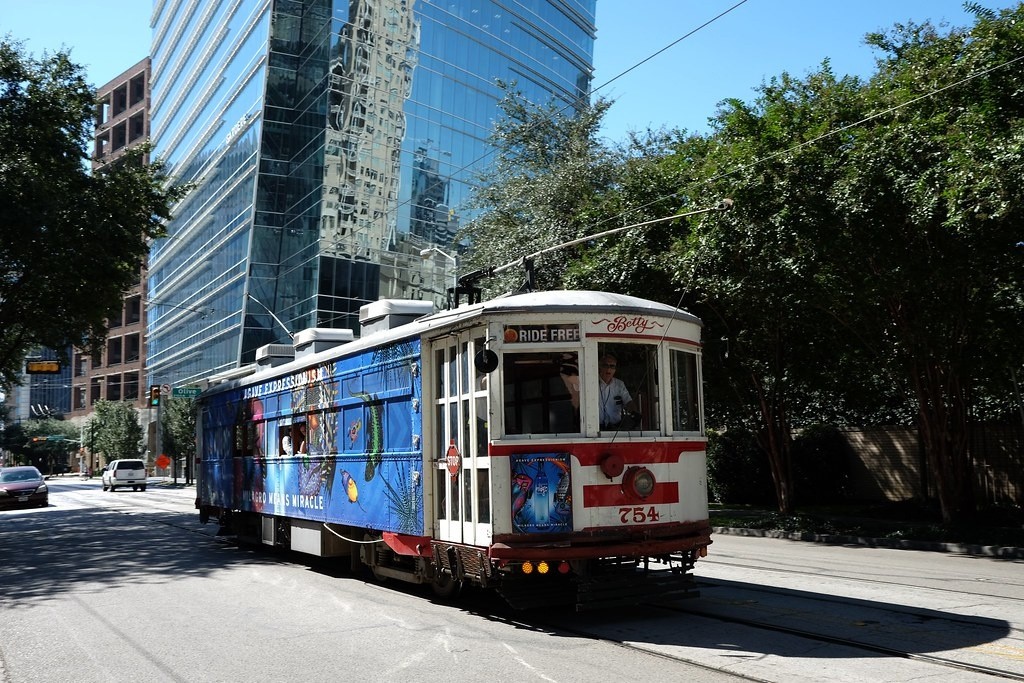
[600,363,616,369]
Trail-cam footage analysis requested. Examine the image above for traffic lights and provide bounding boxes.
[80,447,84,456]
[150,385,160,406]
[38,437,47,440]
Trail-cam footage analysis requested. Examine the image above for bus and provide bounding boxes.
[191,290,714,591]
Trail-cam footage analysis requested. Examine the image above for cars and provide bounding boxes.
[0,466,48,507]
[102,459,146,492]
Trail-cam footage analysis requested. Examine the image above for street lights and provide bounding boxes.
[420,248,457,290]
[33,417,83,478]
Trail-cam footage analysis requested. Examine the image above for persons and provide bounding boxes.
[282,427,292,454]
[297,422,306,454]
[88,467,93,478]
[598,355,638,432]
